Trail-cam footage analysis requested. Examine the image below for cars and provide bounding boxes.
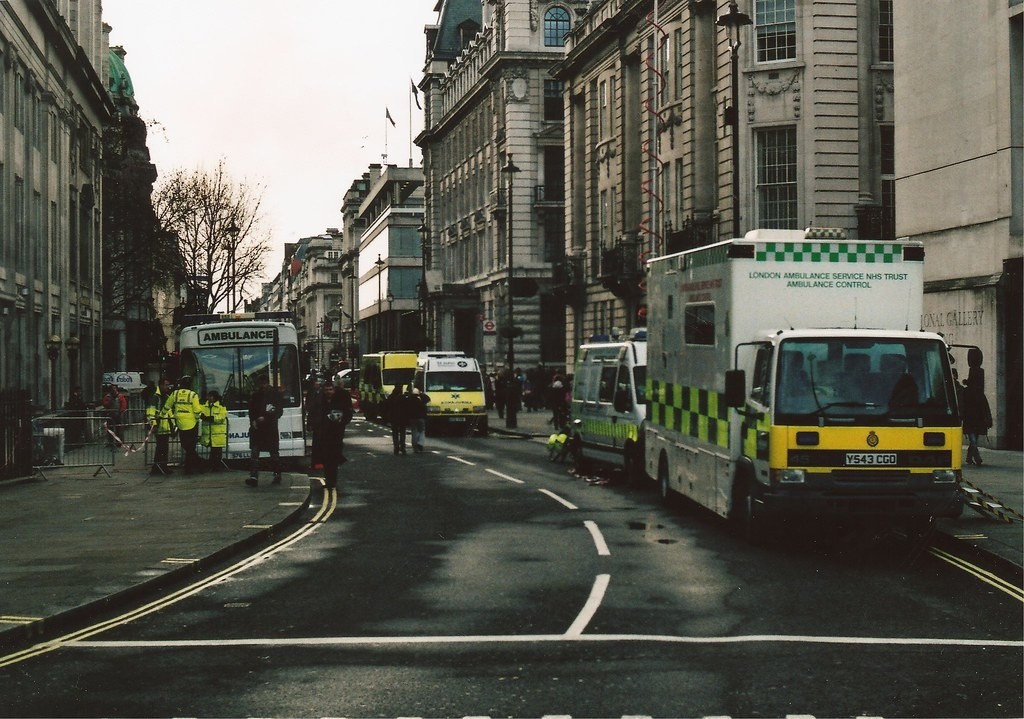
[331,369,362,383]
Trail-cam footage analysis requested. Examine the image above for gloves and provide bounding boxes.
[200,413,214,423]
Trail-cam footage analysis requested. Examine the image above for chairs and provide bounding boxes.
[782,351,807,387]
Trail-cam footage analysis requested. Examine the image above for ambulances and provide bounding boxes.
[645,229,989,554]
[359,351,419,422]
[415,350,491,437]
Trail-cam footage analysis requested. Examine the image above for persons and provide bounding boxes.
[101,384,127,448]
[388,382,431,457]
[245,375,283,487]
[952,368,993,466]
[140,374,228,475]
[478,361,575,430]
[305,378,353,488]
[69,386,95,445]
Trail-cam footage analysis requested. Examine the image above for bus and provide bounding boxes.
[177,312,307,466]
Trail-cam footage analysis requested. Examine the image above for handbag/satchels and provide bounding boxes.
[546,432,570,455]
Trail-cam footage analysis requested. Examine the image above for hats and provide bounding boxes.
[552,380,564,389]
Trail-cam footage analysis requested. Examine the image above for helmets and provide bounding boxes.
[180,375,193,388]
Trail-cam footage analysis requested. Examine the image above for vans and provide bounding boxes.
[572,329,647,479]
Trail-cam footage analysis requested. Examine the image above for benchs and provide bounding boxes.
[844,353,918,407]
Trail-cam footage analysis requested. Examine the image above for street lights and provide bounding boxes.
[375,253,385,353]
[348,272,358,372]
[501,153,526,429]
[316,299,345,372]
[715,0,755,237]
[416,224,436,353]
[225,217,241,313]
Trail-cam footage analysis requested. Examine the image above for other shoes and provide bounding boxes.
[272,471,281,483]
[106,443,114,448]
[245,478,258,487]
[394,445,407,456]
[412,443,423,454]
